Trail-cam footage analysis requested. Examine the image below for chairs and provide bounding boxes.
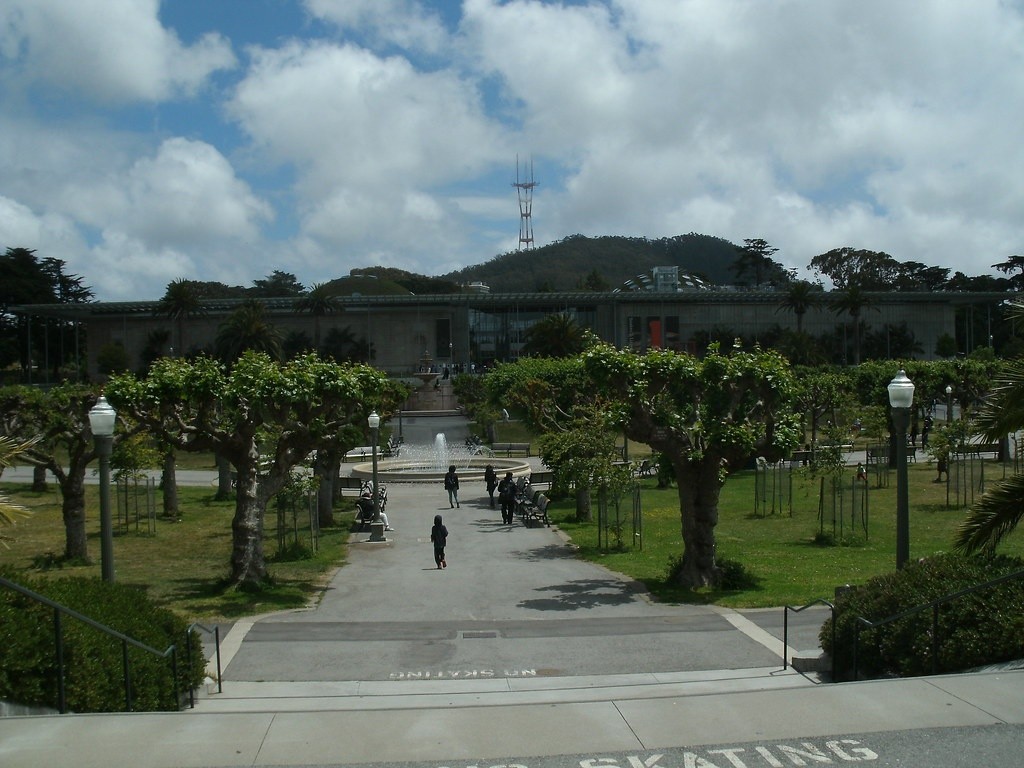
[611,460,653,479]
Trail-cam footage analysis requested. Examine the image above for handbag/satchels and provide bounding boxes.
[448,476,456,488]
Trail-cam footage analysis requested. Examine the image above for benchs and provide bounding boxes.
[387,435,404,458]
[867,446,917,464]
[465,433,483,455]
[815,439,855,453]
[790,451,814,467]
[489,442,532,458]
[357,480,388,531]
[952,442,1001,461]
[229,470,270,490]
[312,445,385,463]
[608,446,628,462]
[306,477,363,497]
[513,475,551,528]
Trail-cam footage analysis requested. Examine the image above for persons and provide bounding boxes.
[442,367,449,380]
[909,425,919,446]
[856,462,868,483]
[498,472,518,524]
[430,515,448,569]
[925,408,933,426]
[356,493,394,530]
[420,365,467,376]
[920,422,931,453]
[802,444,811,466]
[500,407,509,424]
[445,465,460,508]
[856,416,861,430]
[827,419,832,428]
[935,447,947,482]
[485,465,498,507]
[470,363,476,374]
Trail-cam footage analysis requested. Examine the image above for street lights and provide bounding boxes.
[946,385,952,423]
[368,409,387,541]
[89,387,118,586]
[887,359,915,568]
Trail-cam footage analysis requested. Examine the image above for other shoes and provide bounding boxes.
[457,503,459,508]
[385,527,394,531]
[442,560,446,567]
[504,516,507,523]
[451,504,454,508]
[437,565,442,569]
